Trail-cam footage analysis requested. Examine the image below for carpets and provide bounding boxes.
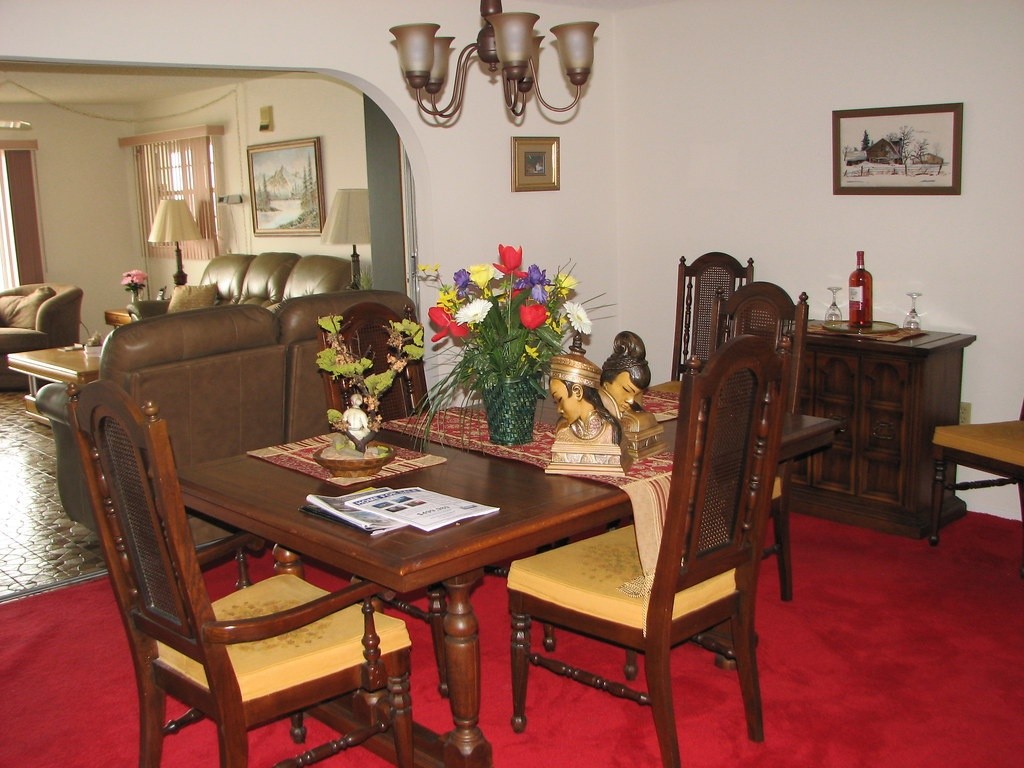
[0,510,1024,767]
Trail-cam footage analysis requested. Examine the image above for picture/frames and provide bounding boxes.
[247,137,326,237]
[832,103,963,195]
[511,136,560,192]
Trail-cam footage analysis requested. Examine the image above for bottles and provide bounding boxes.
[849,251,872,328]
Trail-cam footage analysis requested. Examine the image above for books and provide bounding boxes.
[296,486,500,535]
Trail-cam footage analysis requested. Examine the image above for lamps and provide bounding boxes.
[389,1,599,116]
[321,188,372,290]
[149,199,202,286]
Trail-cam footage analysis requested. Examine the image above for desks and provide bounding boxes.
[7,347,100,427]
[175,380,840,768]
[785,318,977,539]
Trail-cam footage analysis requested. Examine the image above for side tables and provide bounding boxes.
[105,308,131,329]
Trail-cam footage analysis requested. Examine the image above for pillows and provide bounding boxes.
[166,281,218,312]
[0,286,56,330]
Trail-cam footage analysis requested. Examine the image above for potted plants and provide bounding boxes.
[84,332,102,358]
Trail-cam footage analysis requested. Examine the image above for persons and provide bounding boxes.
[546,331,657,442]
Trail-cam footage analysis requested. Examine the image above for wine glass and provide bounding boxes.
[903,293,922,332]
[825,287,842,326]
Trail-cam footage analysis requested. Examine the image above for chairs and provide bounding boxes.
[67,379,414,768]
[0,283,83,390]
[319,252,808,767]
[929,399,1024,579]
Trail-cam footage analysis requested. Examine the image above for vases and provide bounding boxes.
[131,291,139,303]
[313,442,397,477]
[480,371,542,444]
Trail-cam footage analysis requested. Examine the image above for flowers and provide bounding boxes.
[399,244,617,457]
[120,270,148,296]
[316,312,425,453]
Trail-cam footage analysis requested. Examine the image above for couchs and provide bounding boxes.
[125,252,352,319]
[34,289,417,546]
